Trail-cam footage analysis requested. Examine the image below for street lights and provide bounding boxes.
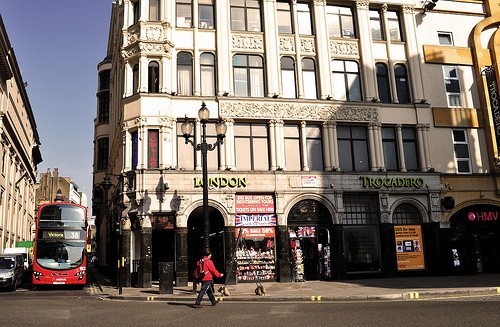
[181,101,228,294]
[100,170,136,295]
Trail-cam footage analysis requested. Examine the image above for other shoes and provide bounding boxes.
[212,301,219,306]
[194,304,202,308]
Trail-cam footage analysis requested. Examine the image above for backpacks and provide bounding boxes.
[194,258,210,285]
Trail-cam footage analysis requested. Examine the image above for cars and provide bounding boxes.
[0,254,25,291]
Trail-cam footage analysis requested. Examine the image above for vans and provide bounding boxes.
[2,247,32,272]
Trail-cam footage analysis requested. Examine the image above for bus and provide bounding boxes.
[29,203,93,286]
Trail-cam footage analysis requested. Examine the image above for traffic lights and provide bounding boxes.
[115,221,122,235]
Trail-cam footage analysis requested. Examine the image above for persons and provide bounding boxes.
[193,248,224,309]
